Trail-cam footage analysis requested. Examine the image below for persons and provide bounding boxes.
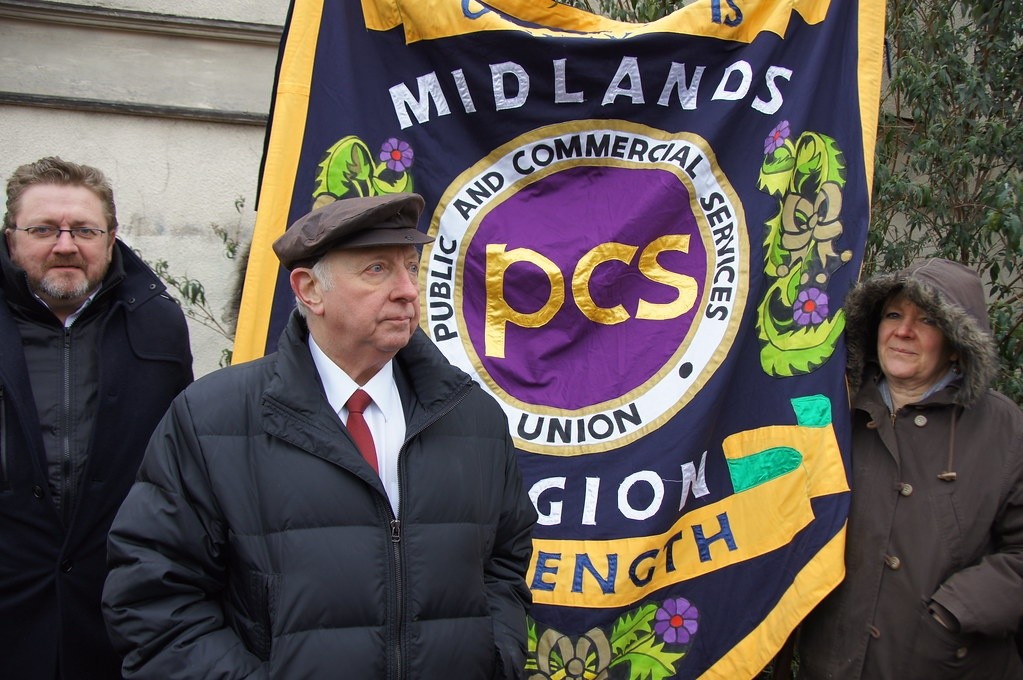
[0,156,194,680]
[100,192,540,680]
[795,256,1023,680]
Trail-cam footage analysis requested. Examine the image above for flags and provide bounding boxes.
[230,0,887,680]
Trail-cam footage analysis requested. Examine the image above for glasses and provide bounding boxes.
[13,226,112,244]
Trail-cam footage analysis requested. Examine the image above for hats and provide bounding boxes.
[272,193,436,273]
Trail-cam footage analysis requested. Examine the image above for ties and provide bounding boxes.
[345,388,379,477]
